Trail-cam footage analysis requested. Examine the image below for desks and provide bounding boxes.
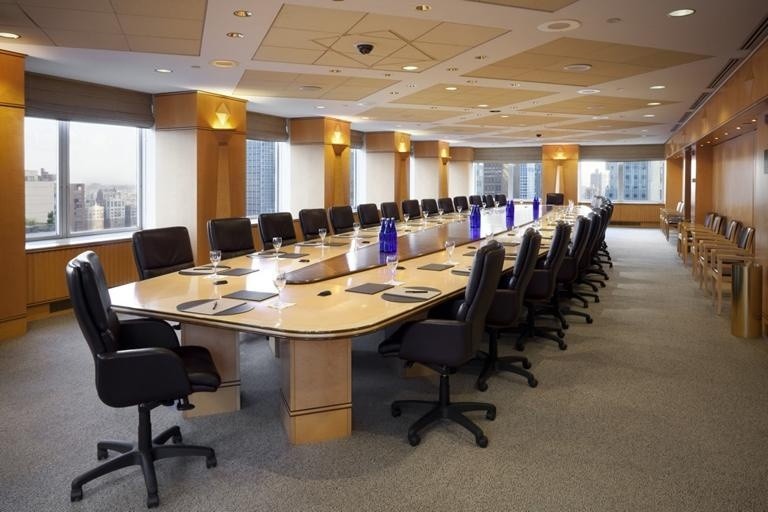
[109,203,592,445]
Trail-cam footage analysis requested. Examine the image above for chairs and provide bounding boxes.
[131,226,195,280]
[207,218,256,259]
[571,187,613,325]
[377,240,506,449]
[495,194,507,206]
[380,202,400,219]
[473,228,542,392]
[421,198,438,213]
[328,206,356,233]
[65,248,221,508]
[454,196,468,210]
[299,208,332,240]
[438,197,455,212]
[469,195,483,207]
[512,222,572,351]
[357,204,381,228]
[659,201,685,241]
[677,212,755,316]
[258,212,297,250]
[547,192,564,204]
[401,200,421,217]
[483,194,494,207]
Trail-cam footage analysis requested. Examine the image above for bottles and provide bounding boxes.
[506,200,514,216]
[378,254,397,265]
[469,229,480,240]
[378,218,397,253]
[533,196,539,210]
[533,210,539,220]
[506,216,514,230]
[470,205,480,229]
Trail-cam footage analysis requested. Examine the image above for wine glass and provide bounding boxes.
[386,255,398,284]
[494,201,499,211]
[445,240,456,264]
[438,209,445,219]
[209,250,221,278]
[404,213,409,224]
[456,206,463,216]
[273,237,282,256]
[422,211,428,221]
[319,229,326,248]
[353,223,360,240]
[208,275,222,304]
[482,202,486,211]
[272,271,286,306]
